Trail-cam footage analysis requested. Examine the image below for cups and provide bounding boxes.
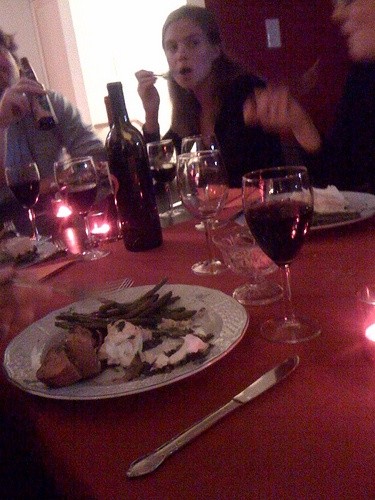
[0,168,36,241]
[182,134,219,152]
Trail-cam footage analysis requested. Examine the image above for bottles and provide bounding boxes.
[107,81,162,251]
[19,56,59,130]
[85,178,121,244]
[104,94,116,133]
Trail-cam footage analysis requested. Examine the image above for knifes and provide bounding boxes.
[121,355,300,478]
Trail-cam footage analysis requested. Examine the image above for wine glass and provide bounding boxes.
[214,226,284,304]
[4,162,52,245]
[147,140,183,233]
[176,150,232,276]
[242,165,321,343]
[54,156,111,261]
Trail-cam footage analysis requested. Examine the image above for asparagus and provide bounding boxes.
[53,276,199,331]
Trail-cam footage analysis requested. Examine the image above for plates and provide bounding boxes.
[305,189,375,230]
[4,284,249,398]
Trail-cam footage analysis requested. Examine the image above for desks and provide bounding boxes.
[0,189,375,500]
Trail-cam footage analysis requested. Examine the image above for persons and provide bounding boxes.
[135,6,286,189]
[243,0,375,196]
[0,29,107,184]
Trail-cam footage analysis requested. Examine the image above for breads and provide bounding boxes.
[35,332,100,389]
